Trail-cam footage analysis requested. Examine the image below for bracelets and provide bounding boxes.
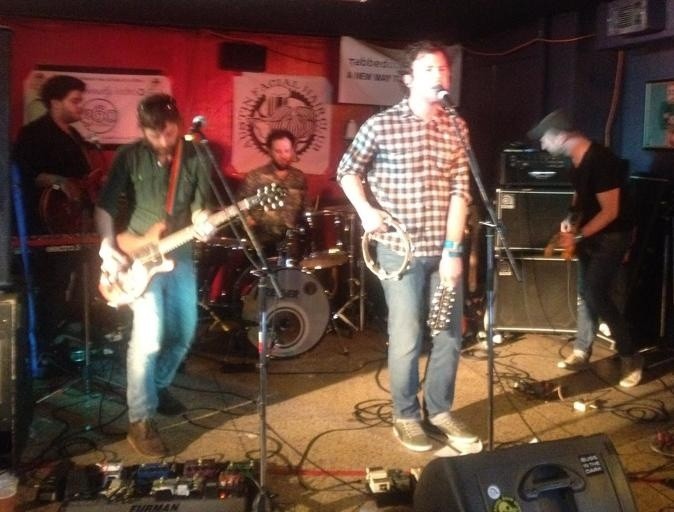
[444,239,465,258]
[574,231,584,243]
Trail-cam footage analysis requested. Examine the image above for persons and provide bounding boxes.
[334,40,485,457]
[525,109,645,389]
[91,93,217,461]
[8,72,97,340]
[659,81,674,147]
[235,128,312,240]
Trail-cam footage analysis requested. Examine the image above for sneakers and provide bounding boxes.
[618,353,645,388]
[392,418,433,452]
[422,410,478,444]
[126,418,168,459]
[556,349,591,371]
[157,388,187,417]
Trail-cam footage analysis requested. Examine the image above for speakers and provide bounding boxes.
[0,289,36,467]
[412,432,640,511]
[219,40,269,72]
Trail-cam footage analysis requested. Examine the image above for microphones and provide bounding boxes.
[183,113,206,144]
[434,84,457,109]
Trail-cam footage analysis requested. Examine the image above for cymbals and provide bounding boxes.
[324,203,356,214]
[329,176,366,184]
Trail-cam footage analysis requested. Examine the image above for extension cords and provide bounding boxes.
[365,464,392,497]
[388,468,411,489]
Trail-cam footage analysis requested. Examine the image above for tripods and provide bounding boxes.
[318,270,359,355]
[35,259,127,432]
[336,215,385,339]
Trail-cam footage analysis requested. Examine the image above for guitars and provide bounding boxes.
[557,206,585,263]
[98,182,289,306]
[39,167,109,235]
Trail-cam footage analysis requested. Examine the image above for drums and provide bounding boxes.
[207,206,255,249]
[229,256,331,359]
[298,248,350,299]
[300,208,348,255]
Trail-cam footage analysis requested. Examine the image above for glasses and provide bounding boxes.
[139,100,175,111]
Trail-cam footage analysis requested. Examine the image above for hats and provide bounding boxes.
[526,108,571,141]
